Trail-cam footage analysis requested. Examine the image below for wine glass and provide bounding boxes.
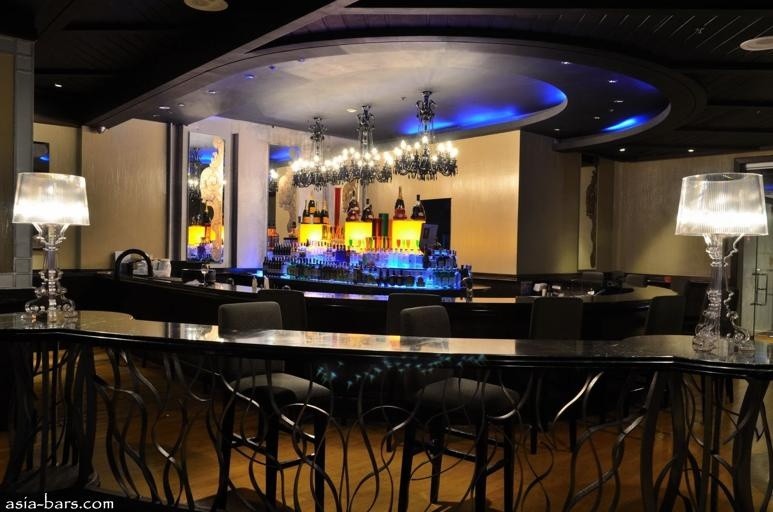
[199,263,209,286]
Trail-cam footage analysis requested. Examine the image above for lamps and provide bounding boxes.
[290,113,339,189]
[330,104,394,185]
[669,166,772,357]
[393,88,460,182]
[7,170,92,330]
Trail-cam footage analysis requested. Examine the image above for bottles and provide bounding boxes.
[268,225,279,249]
[136,257,172,278]
[197,237,209,263]
[262,238,473,291]
[289,222,300,239]
[299,187,427,224]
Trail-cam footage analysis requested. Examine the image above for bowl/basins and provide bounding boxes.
[182,268,216,283]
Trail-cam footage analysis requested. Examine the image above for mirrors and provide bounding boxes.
[185,129,227,265]
[265,140,303,250]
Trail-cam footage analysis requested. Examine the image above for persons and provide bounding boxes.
[283,218,296,245]
